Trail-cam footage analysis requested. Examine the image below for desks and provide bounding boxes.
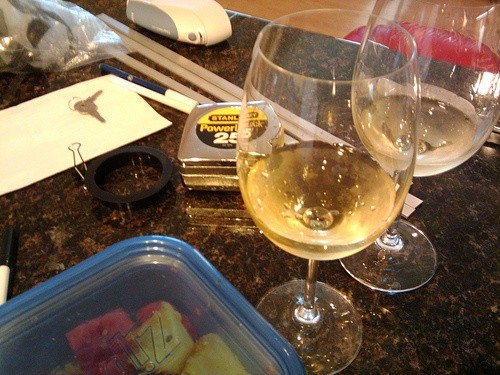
[0,0,500,375]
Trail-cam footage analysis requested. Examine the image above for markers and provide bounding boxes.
[0,223,16,307]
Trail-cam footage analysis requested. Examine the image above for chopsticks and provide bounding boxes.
[95,13,423,218]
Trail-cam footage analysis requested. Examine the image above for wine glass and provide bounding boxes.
[234,9,422,374]
[338,0,499,293]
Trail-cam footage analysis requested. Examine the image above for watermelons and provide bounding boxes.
[66,300,196,375]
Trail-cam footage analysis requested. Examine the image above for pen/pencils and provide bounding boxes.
[110,74,193,115]
[99,62,199,107]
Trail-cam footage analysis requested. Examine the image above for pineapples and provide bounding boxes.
[127,301,249,375]
